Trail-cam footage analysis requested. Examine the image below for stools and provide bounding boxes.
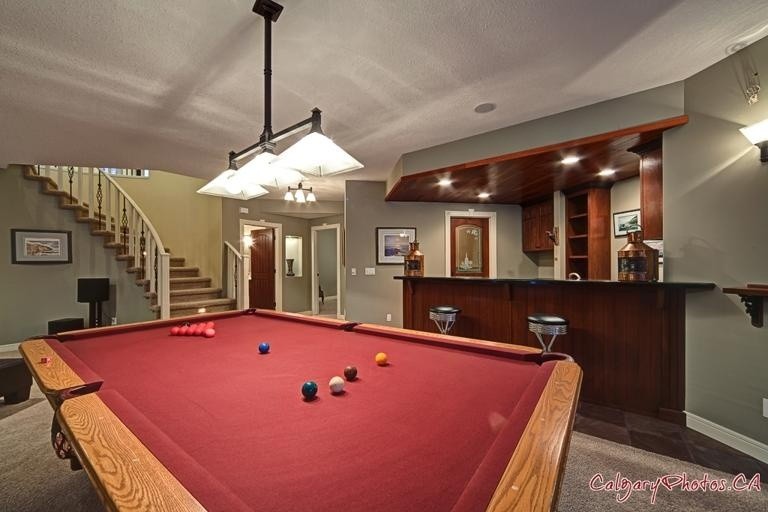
[526,312,568,352]
[428,305,461,335]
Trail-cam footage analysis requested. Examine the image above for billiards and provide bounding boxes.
[259,342,269,352]
[375,351,388,365]
[328,375,344,392]
[302,381,318,399]
[344,366,357,379]
[171,321,215,338]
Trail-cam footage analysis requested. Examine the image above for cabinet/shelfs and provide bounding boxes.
[564,189,611,281]
[521,198,554,253]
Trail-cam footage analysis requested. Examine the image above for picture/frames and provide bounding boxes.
[613,209,642,238]
[10,228,73,265]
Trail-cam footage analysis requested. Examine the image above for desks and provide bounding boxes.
[18,307,585,512]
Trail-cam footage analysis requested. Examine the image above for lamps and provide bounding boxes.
[76,277,109,328]
[375,226,416,265]
[193,0,365,202]
[736,118,767,162]
[284,183,317,203]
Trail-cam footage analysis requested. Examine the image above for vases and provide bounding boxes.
[285,258,295,276]
